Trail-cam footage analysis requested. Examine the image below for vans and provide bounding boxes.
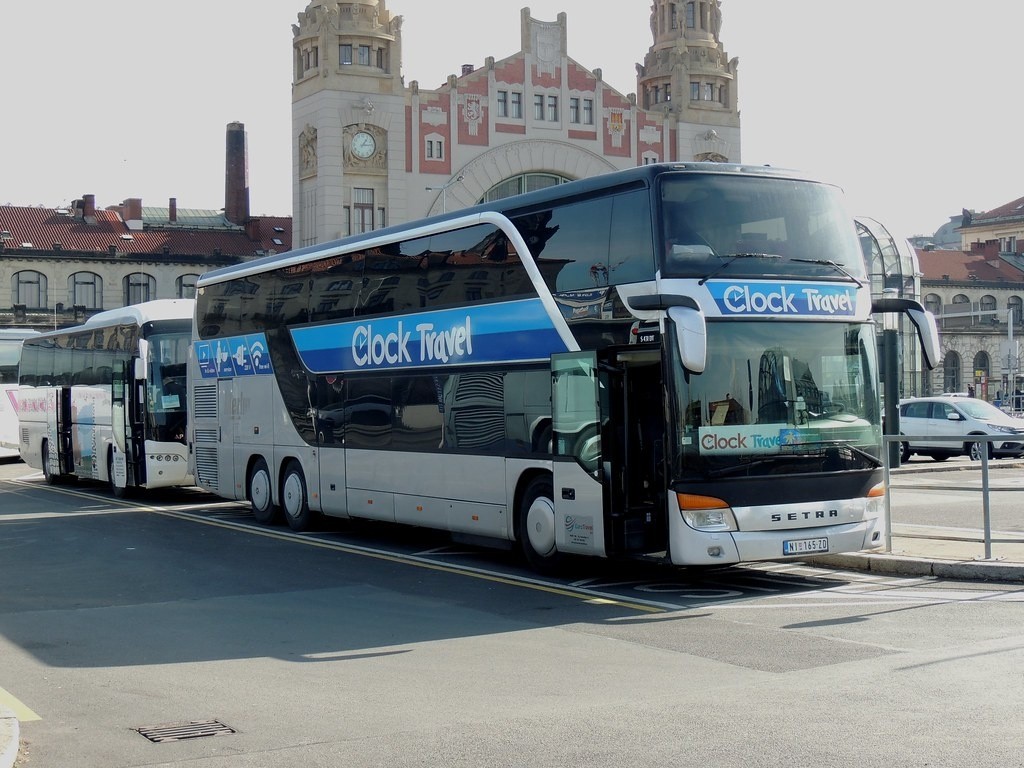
[941,393,970,397]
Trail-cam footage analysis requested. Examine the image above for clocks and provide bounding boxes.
[351,131,376,159]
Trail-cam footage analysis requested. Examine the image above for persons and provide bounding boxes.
[1013,386,1022,411]
[968,384,975,397]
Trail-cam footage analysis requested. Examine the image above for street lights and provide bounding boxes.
[883,287,901,468]
[425,175,464,214]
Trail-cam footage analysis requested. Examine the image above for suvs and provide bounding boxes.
[899,396,1024,463]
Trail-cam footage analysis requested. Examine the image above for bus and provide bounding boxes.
[17,299,257,499]
[186,161,941,578]
[0,329,41,459]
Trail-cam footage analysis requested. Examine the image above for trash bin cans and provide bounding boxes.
[993,400,1001,409]
